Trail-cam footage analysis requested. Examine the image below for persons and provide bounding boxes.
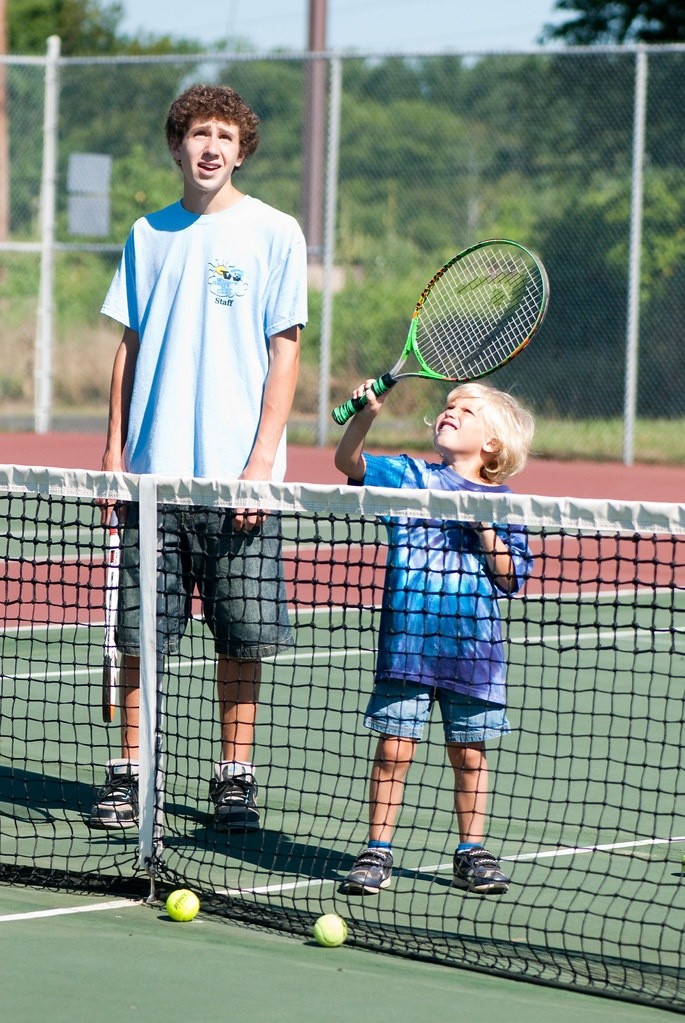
[334,378,533,894]
[88,83,310,828]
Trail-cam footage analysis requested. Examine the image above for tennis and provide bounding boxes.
[167,888,200,923]
[314,912,348,948]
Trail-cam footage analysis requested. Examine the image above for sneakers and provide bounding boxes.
[90,759,140,828]
[453,846,509,893]
[209,773,260,831]
[342,847,394,893]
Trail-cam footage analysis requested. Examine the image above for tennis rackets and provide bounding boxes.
[331,237,550,427]
